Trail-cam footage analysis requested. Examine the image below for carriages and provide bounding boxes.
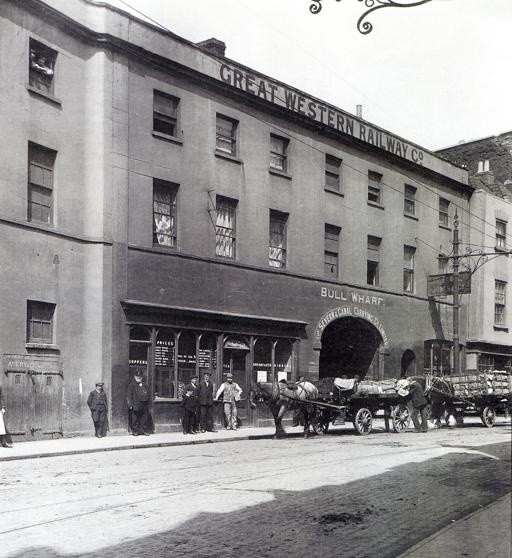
[244,374,413,439]
[403,371,512,427]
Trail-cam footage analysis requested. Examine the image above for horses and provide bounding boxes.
[247,380,319,439]
[405,376,454,429]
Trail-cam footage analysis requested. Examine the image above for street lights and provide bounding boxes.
[437,205,512,373]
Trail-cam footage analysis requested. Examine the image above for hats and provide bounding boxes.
[203,370,213,376]
[189,375,199,379]
[225,373,233,378]
[94,382,105,386]
[134,371,144,377]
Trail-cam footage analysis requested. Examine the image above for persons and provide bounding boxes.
[198,372,218,433]
[403,376,428,433]
[87,381,108,438]
[181,375,199,435]
[271,379,287,439]
[291,375,307,427]
[125,370,150,436]
[0,396,13,448]
[213,372,242,430]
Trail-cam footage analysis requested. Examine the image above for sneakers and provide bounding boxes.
[232,426,236,431]
[226,425,231,431]
[1,442,14,448]
[411,428,429,433]
[133,430,151,436]
[182,429,218,436]
[95,432,106,438]
